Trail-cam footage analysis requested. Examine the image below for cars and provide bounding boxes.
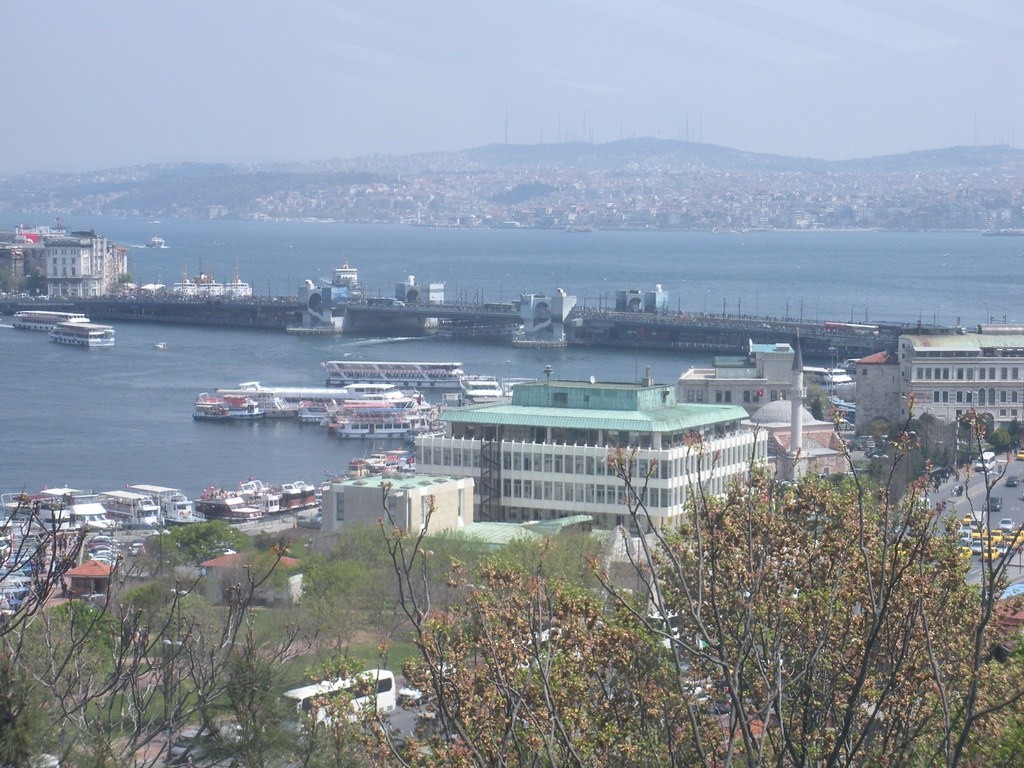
[945,511,1024,562]
[1016,451,1024,460]
[838,418,855,431]
[1006,475,1020,488]
[167,729,214,764]
[998,518,1016,533]
[990,496,1004,512]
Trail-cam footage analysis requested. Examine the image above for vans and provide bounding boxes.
[975,452,996,470]
[398,661,459,706]
[914,497,933,511]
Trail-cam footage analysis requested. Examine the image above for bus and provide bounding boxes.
[824,323,880,336]
[279,668,395,736]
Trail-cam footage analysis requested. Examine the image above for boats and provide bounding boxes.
[145,236,165,249]
[47,322,116,347]
[1,449,414,610]
[11,310,90,332]
[322,360,465,390]
[196,374,536,445]
[120,255,254,298]
